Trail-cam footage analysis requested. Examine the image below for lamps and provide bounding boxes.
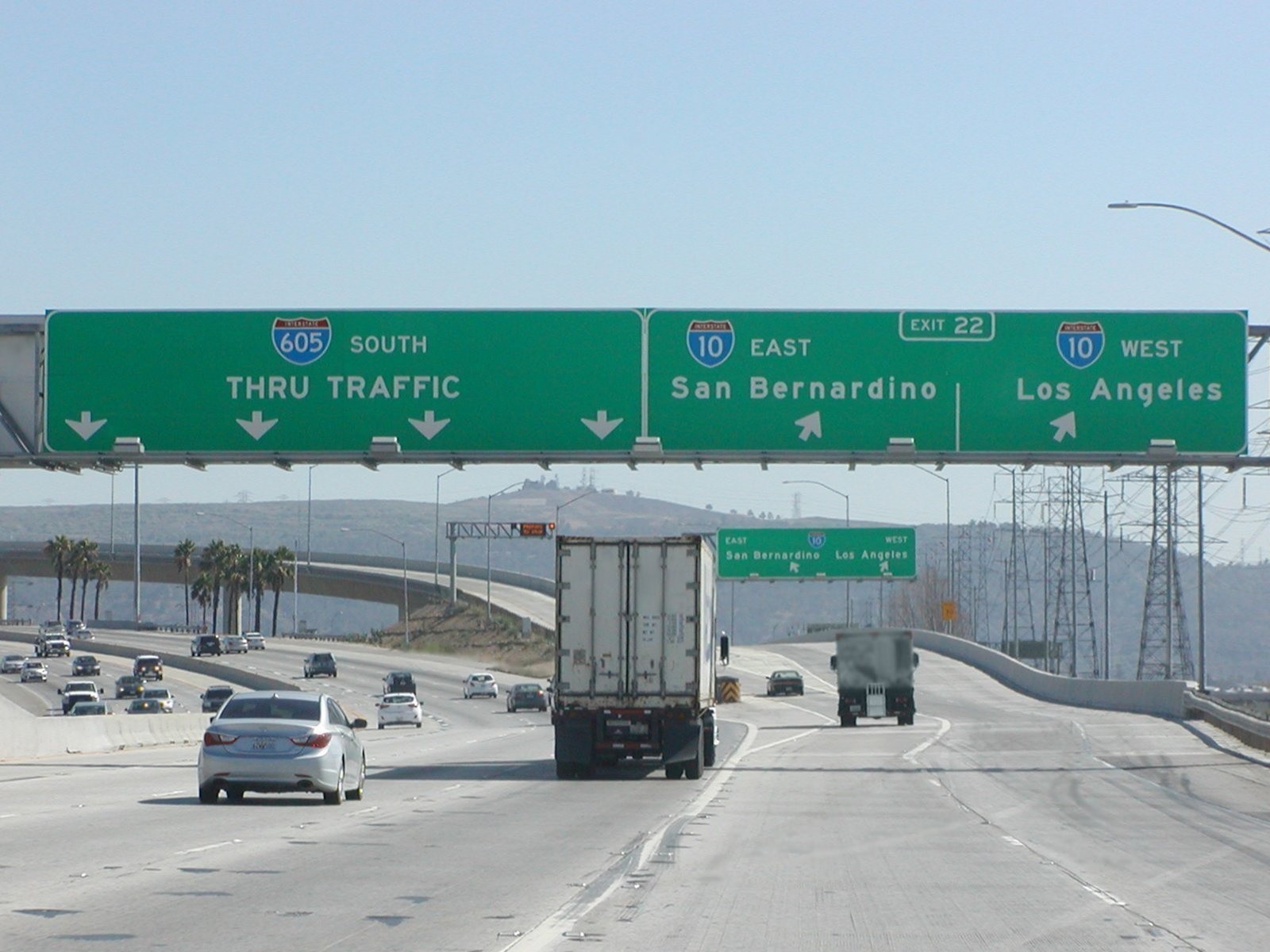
[369,436,400,453]
[1147,439,1177,457]
[886,437,915,454]
[114,437,145,453]
[631,437,664,458]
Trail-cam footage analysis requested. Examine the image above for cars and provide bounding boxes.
[124,700,164,714]
[66,701,115,716]
[766,670,804,696]
[544,673,556,724]
[505,683,547,712]
[190,632,266,657]
[303,652,337,678]
[462,672,498,699]
[383,672,416,695]
[137,687,175,713]
[375,693,425,730]
[0,619,163,716]
[198,690,368,805]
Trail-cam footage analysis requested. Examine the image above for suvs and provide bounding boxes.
[200,685,234,712]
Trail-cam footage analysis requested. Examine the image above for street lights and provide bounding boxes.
[781,479,849,627]
[195,511,253,632]
[339,527,409,644]
[487,480,539,624]
[556,487,615,534]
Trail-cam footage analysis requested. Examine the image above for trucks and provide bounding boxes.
[554,531,731,782]
[830,628,920,727]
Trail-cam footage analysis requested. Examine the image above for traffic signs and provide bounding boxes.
[644,306,958,454]
[956,309,1248,455]
[715,526,918,581]
[40,308,646,454]
